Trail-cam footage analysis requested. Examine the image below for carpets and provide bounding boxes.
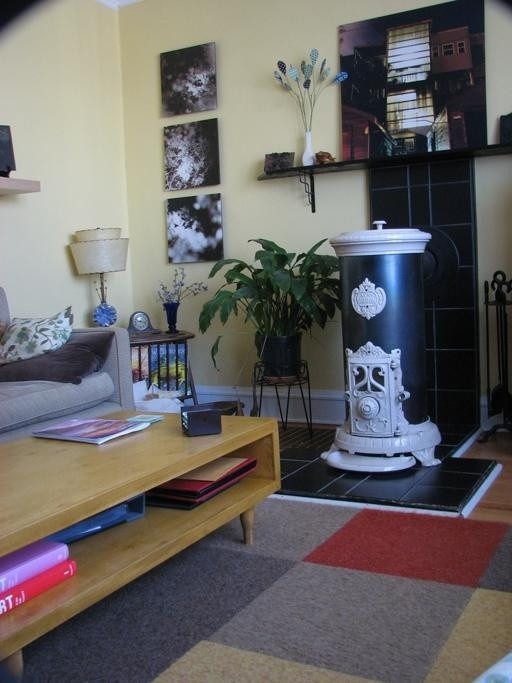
[0,497,511,682]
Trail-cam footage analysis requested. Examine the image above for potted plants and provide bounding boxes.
[198,237,339,384]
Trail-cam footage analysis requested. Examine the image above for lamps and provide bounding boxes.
[69,227,130,327]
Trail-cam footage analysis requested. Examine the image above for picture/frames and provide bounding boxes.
[163,118,220,192]
[165,192,224,264]
[159,42,218,118]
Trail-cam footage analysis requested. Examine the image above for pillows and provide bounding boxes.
[0,304,73,364]
[0,330,115,384]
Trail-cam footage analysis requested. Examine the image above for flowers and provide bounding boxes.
[155,267,208,302]
[273,48,348,131]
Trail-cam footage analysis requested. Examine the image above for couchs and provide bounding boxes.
[0,286,136,441]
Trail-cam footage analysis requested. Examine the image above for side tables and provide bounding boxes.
[130,330,200,407]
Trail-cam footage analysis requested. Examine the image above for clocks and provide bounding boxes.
[127,310,161,334]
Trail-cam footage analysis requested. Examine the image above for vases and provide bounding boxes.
[302,131,316,166]
[163,302,180,333]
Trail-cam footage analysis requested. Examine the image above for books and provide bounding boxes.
[130,342,187,391]
[32,416,151,445]
[0,540,79,615]
[149,457,259,509]
